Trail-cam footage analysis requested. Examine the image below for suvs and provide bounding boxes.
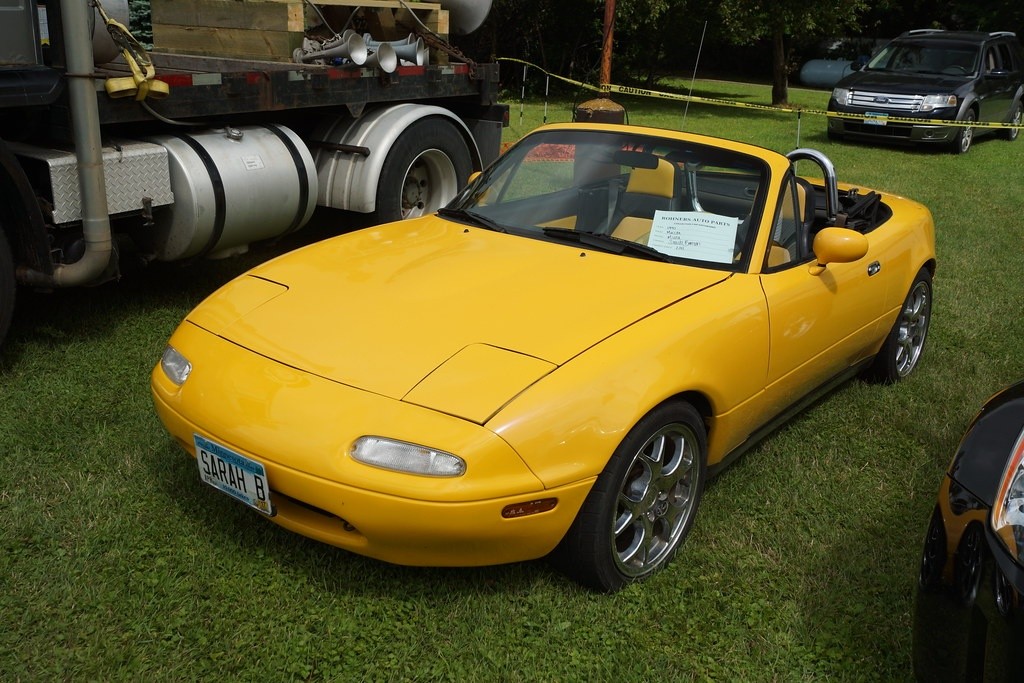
[826,28,1024,155]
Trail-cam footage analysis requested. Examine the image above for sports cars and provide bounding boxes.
[153,123,937,594]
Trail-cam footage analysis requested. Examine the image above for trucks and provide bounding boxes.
[0,0,512,342]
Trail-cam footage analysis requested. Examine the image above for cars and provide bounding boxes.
[917,378,1024,683]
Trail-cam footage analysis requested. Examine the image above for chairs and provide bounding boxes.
[733,176,816,268]
[937,54,972,75]
[606,155,683,247]
